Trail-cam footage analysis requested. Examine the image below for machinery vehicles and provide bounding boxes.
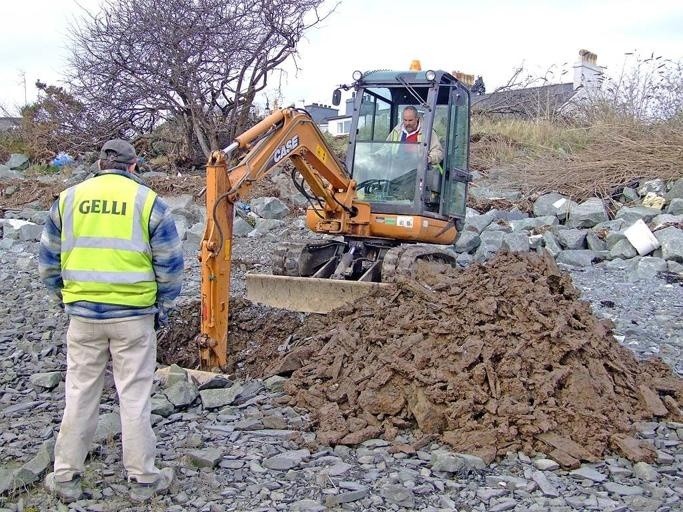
[196,59,472,370]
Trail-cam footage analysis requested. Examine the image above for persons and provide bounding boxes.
[38,139,184,504]
[369,106,444,200]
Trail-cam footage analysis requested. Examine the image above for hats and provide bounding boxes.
[99,139,140,173]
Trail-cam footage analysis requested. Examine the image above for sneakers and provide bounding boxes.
[130,467,174,503]
[44,472,83,503]
